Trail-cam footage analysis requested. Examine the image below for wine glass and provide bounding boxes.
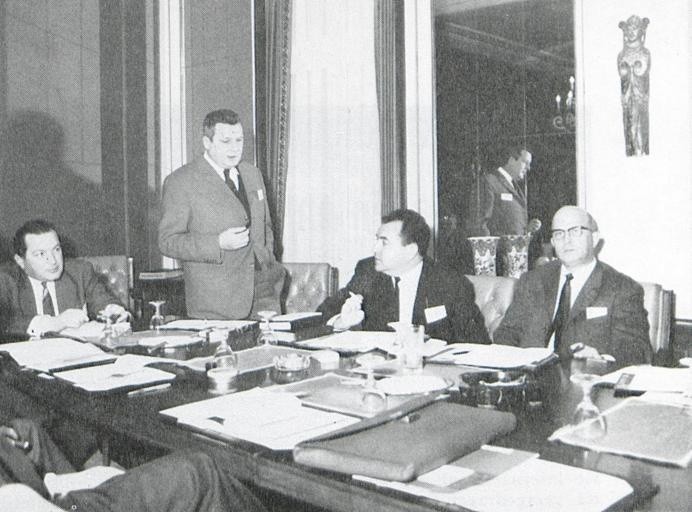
[210,323,236,374]
[257,310,278,351]
[149,301,166,329]
[568,374,606,441]
[354,354,388,415]
[99,310,119,347]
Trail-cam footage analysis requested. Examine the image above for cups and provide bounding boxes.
[399,325,425,375]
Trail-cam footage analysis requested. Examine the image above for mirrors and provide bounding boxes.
[432,0,576,276]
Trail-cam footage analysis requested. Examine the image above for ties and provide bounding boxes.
[223,168,238,196]
[552,273,575,352]
[40,281,55,317]
[511,179,526,203]
[393,276,399,321]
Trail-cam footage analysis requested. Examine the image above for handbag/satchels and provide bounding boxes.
[292,395,517,483]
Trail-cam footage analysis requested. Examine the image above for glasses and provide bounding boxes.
[551,225,593,241]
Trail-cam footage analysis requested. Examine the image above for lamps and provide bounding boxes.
[551,76,577,135]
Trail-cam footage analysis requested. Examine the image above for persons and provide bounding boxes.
[0,220,135,336]
[472,146,541,235]
[492,205,654,367]
[615,15,652,158]
[155,108,291,321]
[317,209,492,343]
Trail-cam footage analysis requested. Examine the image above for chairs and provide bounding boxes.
[642,283,675,355]
[274,263,336,314]
[464,274,520,342]
[73,255,136,317]
